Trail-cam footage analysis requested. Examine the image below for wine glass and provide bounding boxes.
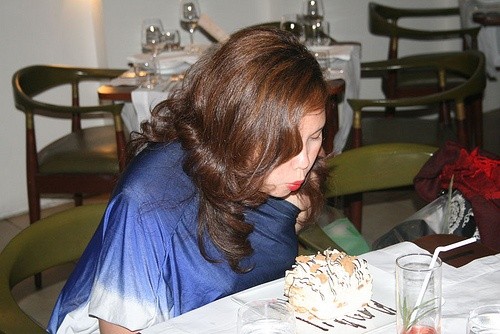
[178,1,200,46]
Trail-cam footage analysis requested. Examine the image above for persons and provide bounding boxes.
[46,24,338,334]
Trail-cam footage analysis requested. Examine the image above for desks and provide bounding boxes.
[98,44,363,230]
[136,233,500,334]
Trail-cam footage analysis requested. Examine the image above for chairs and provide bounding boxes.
[0,0,500,334]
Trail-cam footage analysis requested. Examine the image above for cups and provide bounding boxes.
[280,0,332,83]
[142,20,181,57]
[396,253,443,334]
[238,299,296,334]
[134,57,162,89]
[466,304,500,334]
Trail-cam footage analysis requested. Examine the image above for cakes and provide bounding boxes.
[284,247,374,320]
